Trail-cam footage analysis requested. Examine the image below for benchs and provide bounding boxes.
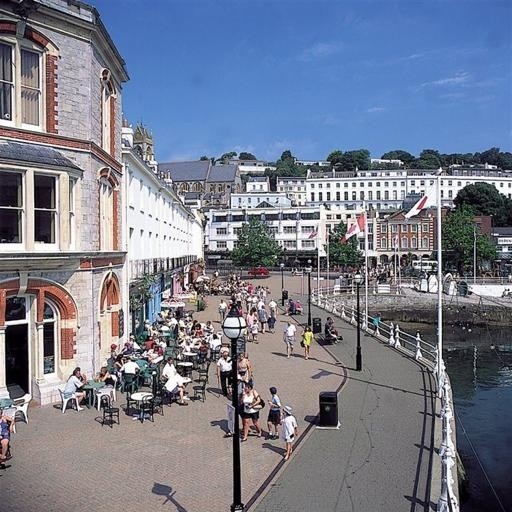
[318,333,334,345]
[290,309,297,315]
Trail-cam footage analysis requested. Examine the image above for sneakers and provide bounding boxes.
[224,430,262,442]
[179,402,188,405]
[264,434,279,440]
[71,403,84,410]
[284,449,292,459]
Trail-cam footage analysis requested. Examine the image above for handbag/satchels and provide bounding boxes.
[252,389,265,409]
[239,371,246,376]
[300,341,306,347]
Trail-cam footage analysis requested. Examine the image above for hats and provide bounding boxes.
[283,406,293,415]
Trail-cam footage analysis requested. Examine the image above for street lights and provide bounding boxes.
[304,263,313,327]
[280,262,285,290]
[353,268,364,371]
[221,293,247,512]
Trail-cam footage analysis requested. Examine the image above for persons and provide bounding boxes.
[285,298,304,316]
[194,271,277,343]
[284,320,296,359]
[301,325,313,360]
[215,346,299,460]
[63,310,222,411]
[0,408,14,459]
[324,317,342,344]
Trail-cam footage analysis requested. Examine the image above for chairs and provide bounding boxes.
[2,391,32,469]
[56,282,252,429]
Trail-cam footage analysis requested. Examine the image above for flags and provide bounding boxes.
[405,181,438,218]
[340,213,364,244]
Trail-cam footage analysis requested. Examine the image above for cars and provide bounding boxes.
[248,267,269,275]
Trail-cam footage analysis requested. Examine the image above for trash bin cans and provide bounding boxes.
[312,317,321,334]
[283,290,288,299]
[319,392,338,427]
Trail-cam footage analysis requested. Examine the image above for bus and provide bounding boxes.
[412,261,438,272]
